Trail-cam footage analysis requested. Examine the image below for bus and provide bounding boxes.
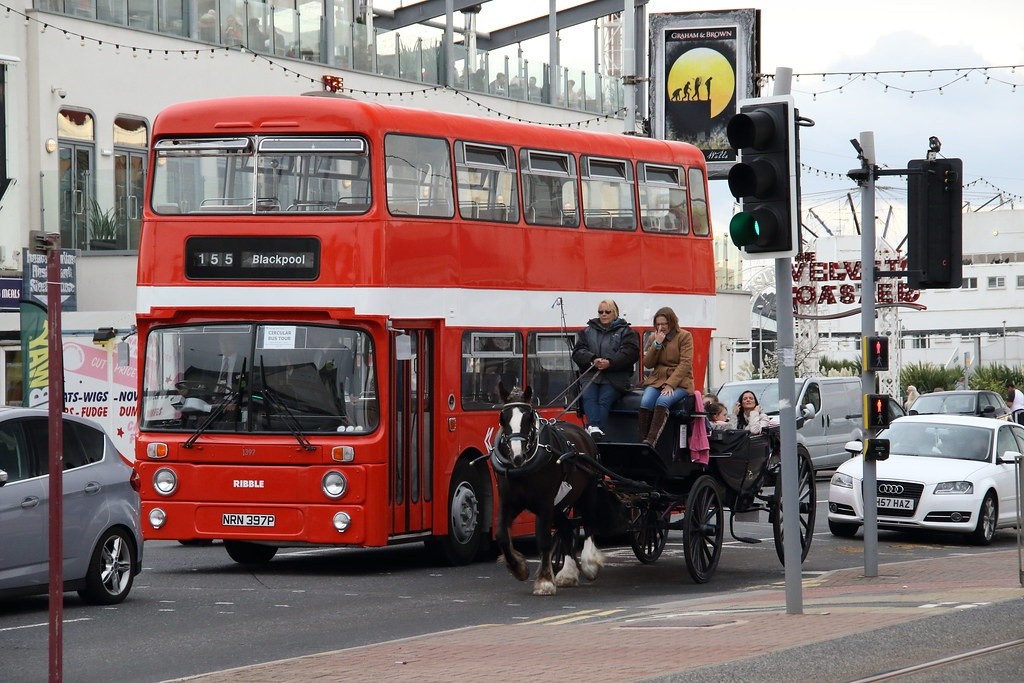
[116,90,717,570]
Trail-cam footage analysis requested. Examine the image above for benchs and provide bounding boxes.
[575,383,693,444]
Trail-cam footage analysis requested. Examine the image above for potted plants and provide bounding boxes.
[399,38,440,83]
[84,196,129,250]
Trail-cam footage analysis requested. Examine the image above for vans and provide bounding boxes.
[715,376,862,471]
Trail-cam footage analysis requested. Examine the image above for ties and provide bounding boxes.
[216,357,228,400]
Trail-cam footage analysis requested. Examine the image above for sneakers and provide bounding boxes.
[587,425,605,439]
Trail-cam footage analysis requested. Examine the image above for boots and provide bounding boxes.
[638,406,653,443]
[643,405,670,448]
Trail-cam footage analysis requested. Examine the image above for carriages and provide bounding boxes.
[469,378,817,595]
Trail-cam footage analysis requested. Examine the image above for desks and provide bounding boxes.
[74,6,320,61]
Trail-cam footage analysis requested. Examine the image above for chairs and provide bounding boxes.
[158,191,686,232]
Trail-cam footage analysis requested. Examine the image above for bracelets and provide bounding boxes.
[653,341,663,349]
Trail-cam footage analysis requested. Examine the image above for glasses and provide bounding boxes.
[655,323,668,327]
[598,310,612,314]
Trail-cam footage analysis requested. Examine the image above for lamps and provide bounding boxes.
[92,328,117,347]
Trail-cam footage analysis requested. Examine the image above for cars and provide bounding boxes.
[0,404,143,607]
[828,414,1024,548]
[906,389,1013,421]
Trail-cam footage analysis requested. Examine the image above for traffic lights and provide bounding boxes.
[862,393,889,429]
[861,334,890,372]
[724,95,800,260]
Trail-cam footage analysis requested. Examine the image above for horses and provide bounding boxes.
[491,381,606,595]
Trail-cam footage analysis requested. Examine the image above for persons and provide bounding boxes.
[904,385,920,413]
[736,391,768,434]
[248,18,269,51]
[199,9,216,23]
[639,306,695,449]
[572,297,640,441]
[702,394,719,408]
[282,330,361,395]
[954,377,972,390]
[489,72,542,103]
[558,80,580,110]
[180,332,249,421]
[704,402,741,431]
[1006,383,1024,410]
[225,15,243,38]
[367,44,382,72]
[454,67,485,92]
[266,26,284,56]
